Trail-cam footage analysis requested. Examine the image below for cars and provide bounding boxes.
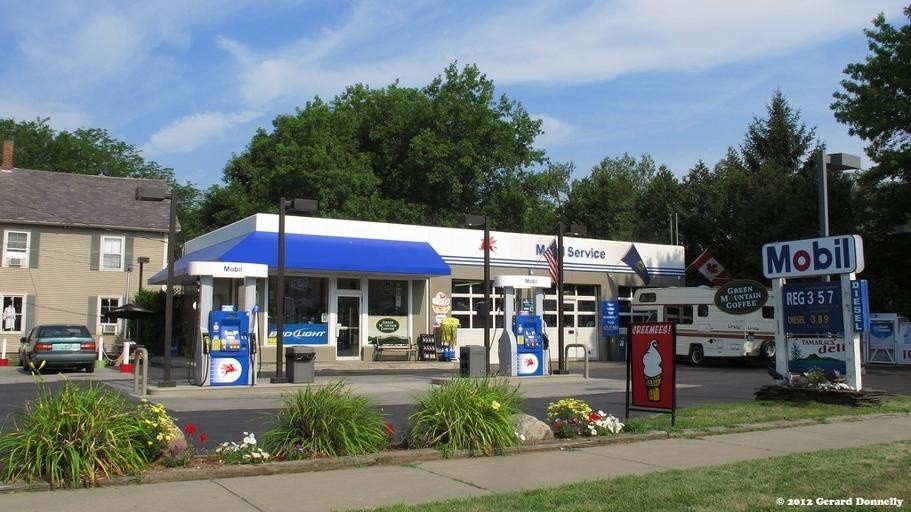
[171,336,185,357]
[19,324,99,373]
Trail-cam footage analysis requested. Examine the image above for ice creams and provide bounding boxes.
[643,340,663,401]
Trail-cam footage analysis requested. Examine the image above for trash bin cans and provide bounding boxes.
[460,345,486,378]
[285,346,316,384]
[608,334,626,361]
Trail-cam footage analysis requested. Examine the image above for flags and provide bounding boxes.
[544,241,558,287]
[696,254,735,287]
[623,245,651,286]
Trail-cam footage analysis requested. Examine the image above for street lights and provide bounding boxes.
[135,187,176,387]
[271,197,318,383]
[817,150,861,338]
[137,257,150,290]
[457,214,490,375]
[553,224,586,374]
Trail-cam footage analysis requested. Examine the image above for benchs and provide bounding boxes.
[373,335,418,362]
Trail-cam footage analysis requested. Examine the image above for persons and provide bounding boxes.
[3,304,17,331]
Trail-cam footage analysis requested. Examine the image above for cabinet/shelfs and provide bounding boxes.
[420,334,437,361]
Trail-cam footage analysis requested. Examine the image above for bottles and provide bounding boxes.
[230,344,239,351]
[213,322,219,340]
[517,324,524,344]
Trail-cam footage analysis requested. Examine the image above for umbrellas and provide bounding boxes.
[103,304,153,340]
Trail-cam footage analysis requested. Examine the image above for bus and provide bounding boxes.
[631,285,775,366]
